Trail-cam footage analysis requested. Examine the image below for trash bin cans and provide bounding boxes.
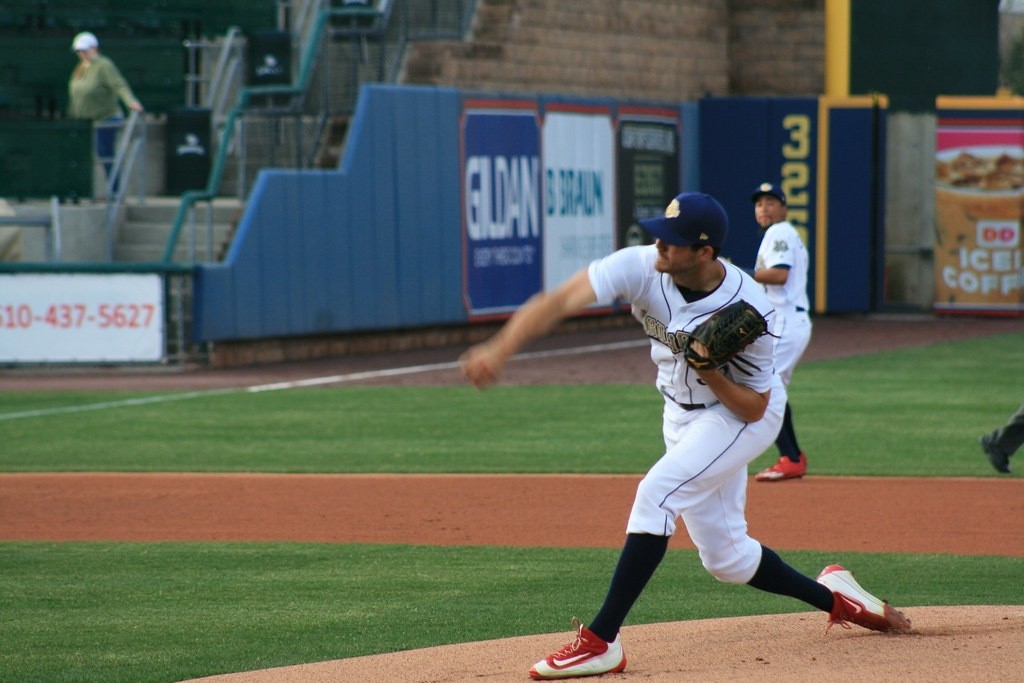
[162,107,213,198]
[241,29,294,110]
[328,0,383,43]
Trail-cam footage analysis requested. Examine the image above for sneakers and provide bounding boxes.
[755,452,807,481]
[816,564,912,636]
[529,616,627,679]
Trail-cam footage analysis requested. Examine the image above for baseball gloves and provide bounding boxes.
[684,297,771,367]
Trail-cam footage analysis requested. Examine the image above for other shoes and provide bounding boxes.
[980,428,1009,474]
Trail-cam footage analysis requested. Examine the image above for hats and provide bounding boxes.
[639,192,728,249]
[72,31,98,51]
[751,182,786,206]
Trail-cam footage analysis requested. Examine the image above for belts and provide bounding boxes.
[666,393,705,411]
[796,306,804,312]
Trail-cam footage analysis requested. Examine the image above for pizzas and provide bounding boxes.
[934,151,1024,191]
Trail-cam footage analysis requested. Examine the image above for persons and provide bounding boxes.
[67,30,143,203]
[739,184,814,482]
[456,190,914,679]
[979,404,1024,473]
